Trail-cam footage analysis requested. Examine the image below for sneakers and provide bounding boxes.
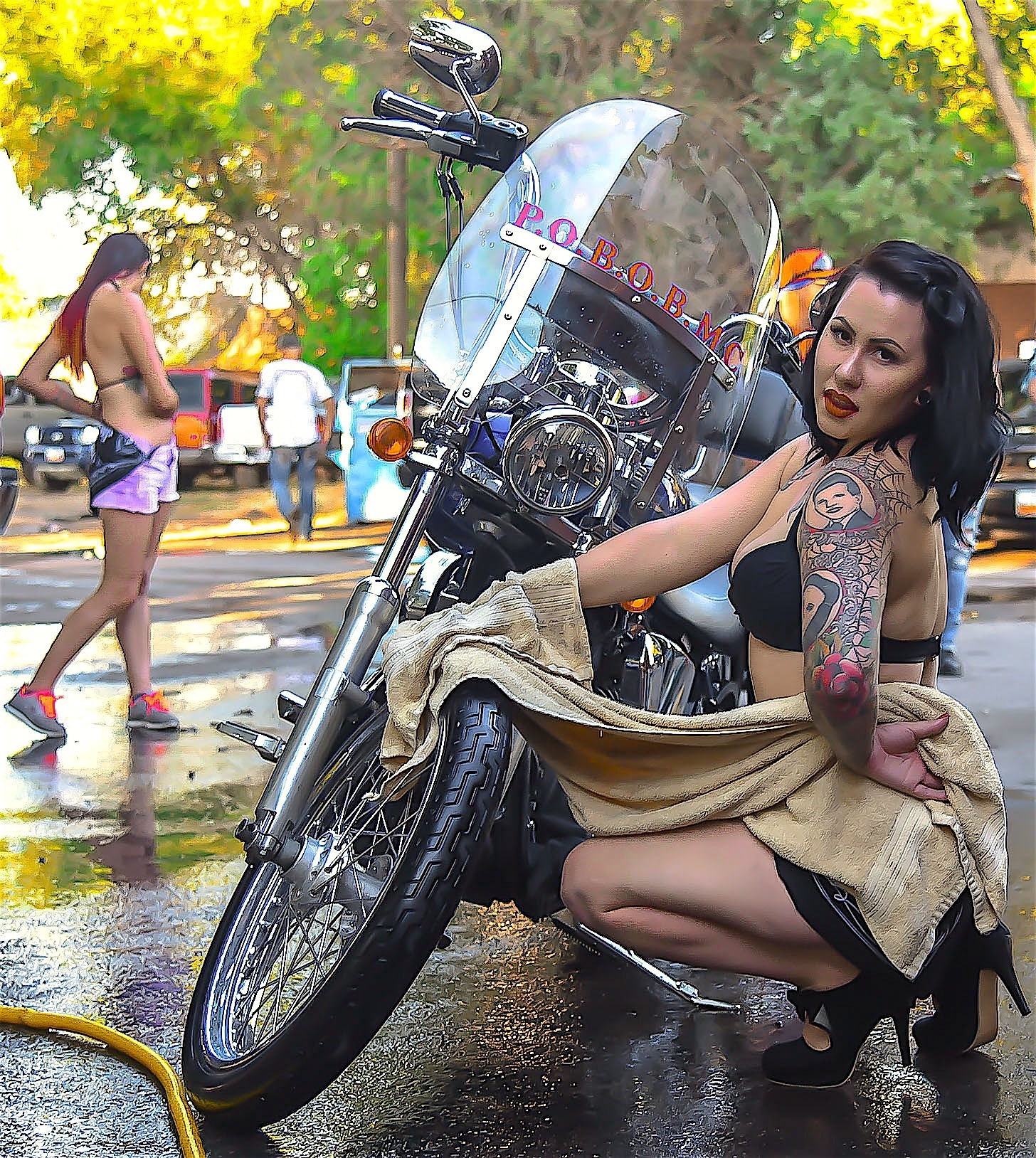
[129,728,179,756]
[127,688,179,729]
[7,737,66,775]
[4,683,65,737]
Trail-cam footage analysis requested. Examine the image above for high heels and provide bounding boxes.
[913,891,1032,1053]
[760,970,916,1089]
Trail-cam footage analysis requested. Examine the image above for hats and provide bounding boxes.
[276,334,299,348]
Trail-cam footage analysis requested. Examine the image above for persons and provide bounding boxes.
[255,334,335,542]
[934,481,990,674]
[4,234,181,739]
[381,239,1031,1089]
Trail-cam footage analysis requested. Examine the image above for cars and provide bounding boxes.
[21,417,105,493]
[1,375,77,483]
[977,339,1036,527]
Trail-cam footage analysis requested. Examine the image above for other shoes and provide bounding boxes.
[938,650,962,676]
[300,523,315,538]
[290,507,303,539]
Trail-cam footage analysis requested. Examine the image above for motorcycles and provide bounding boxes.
[179,18,840,1131]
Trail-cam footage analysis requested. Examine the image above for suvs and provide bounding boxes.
[165,369,270,492]
